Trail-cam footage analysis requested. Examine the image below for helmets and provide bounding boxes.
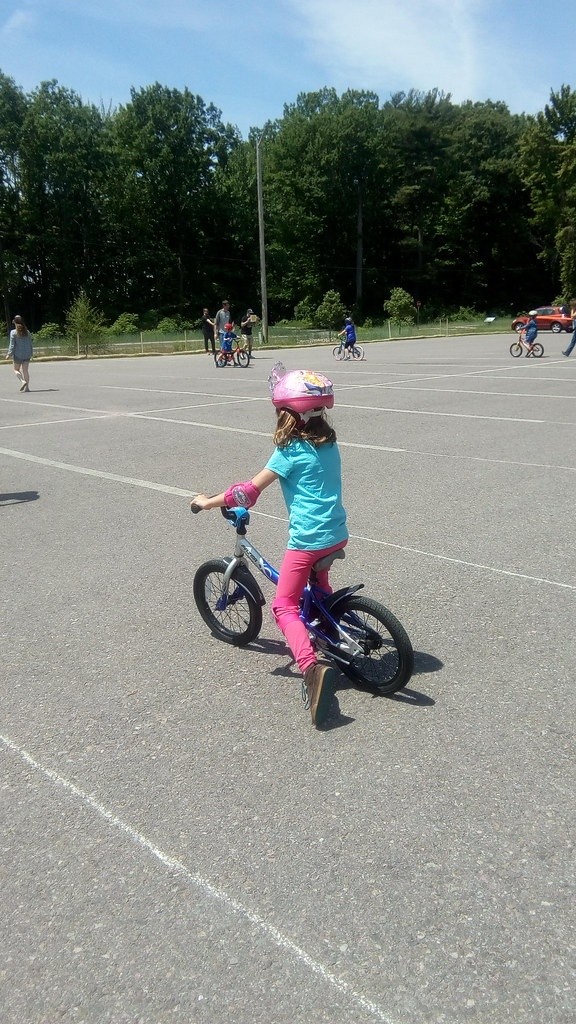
[273,369,334,412]
[224,323,232,329]
[529,310,537,316]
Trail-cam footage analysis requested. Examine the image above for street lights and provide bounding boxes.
[255,122,276,344]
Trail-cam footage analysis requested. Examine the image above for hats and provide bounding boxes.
[248,309,254,314]
[346,318,352,322]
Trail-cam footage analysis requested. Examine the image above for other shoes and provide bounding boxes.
[234,363,239,366]
[525,350,533,358]
[344,356,349,361]
[219,354,223,361]
[249,355,255,359]
[562,350,568,356]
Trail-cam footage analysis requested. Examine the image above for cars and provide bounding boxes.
[511,306,574,334]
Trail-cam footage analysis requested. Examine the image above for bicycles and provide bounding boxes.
[191,493,414,694]
[214,338,250,368]
[510,329,544,358]
[333,336,364,361]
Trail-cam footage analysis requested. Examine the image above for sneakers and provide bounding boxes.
[310,635,335,649]
[301,662,333,727]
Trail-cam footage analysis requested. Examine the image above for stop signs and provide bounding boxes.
[417,301,421,307]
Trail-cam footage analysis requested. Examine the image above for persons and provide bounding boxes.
[562,301,576,357]
[202,308,220,355]
[516,310,538,357]
[6,315,33,392]
[215,323,239,367]
[191,360,349,725]
[240,309,260,359]
[338,318,356,360]
[214,301,231,350]
